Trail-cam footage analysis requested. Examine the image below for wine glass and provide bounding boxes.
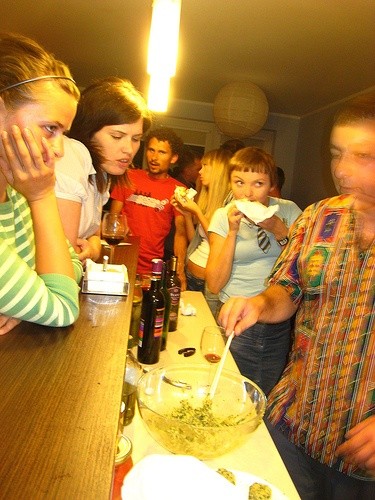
[102,213,128,264]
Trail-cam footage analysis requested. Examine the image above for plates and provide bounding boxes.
[229,469,288,500]
[121,454,247,500]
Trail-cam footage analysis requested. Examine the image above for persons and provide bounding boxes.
[218,97,375,500]
[50,76,152,270]
[205,146,304,399]
[93,128,189,292]
[0,31,83,335]
[162,137,286,314]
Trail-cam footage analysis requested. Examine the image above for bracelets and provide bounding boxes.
[276,237,289,247]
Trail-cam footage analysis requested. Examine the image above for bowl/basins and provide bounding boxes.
[136,363,267,461]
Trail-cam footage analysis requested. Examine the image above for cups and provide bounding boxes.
[200,325,228,363]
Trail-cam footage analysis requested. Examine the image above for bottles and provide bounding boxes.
[127,256,181,365]
